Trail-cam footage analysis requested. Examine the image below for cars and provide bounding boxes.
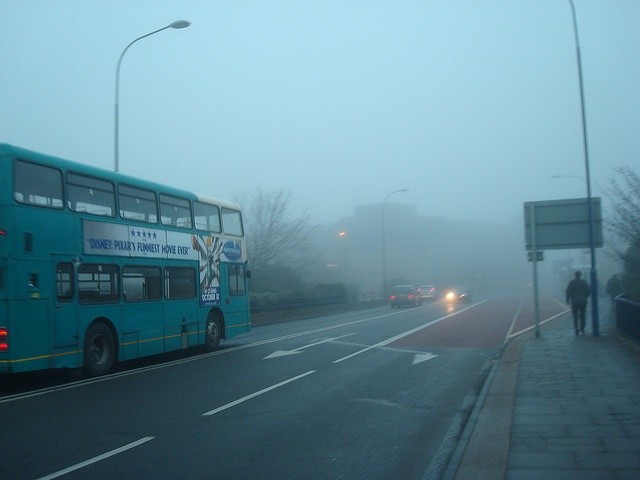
[446,287,473,304]
[390,284,421,308]
[417,285,440,303]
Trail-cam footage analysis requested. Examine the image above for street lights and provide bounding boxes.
[380,188,410,299]
[113,19,191,174]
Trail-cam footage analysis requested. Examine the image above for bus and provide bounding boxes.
[0,142,253,379]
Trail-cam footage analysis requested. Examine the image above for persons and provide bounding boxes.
[565,270,591,336]
[606,274,620,312]
